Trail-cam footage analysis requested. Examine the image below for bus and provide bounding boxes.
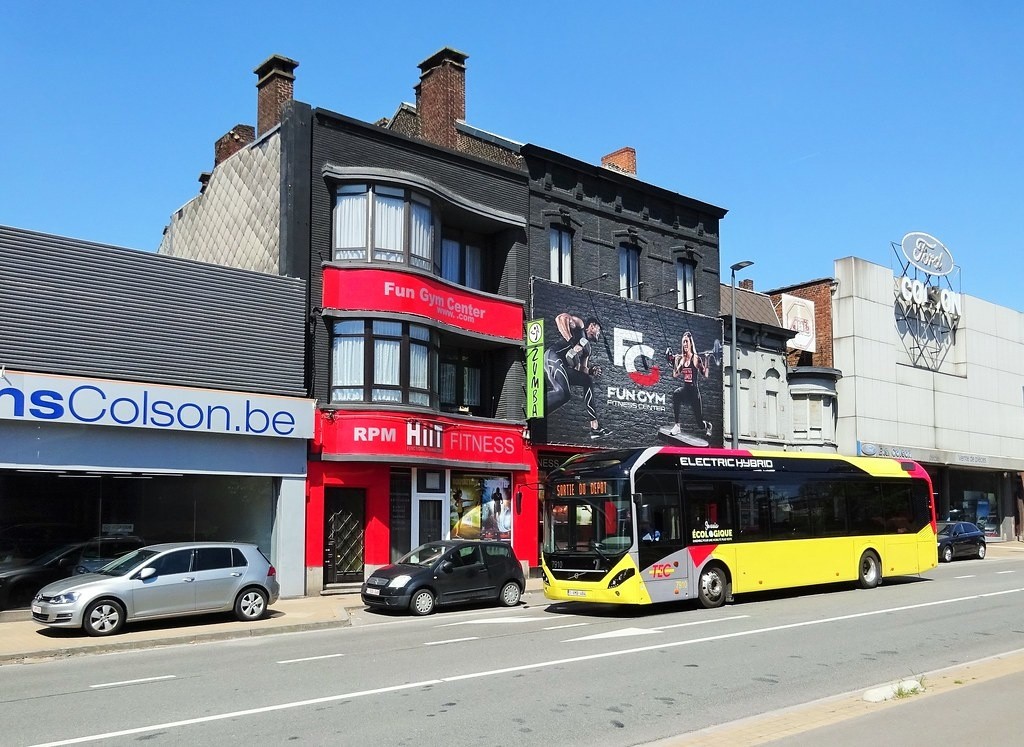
[516,445,938,608]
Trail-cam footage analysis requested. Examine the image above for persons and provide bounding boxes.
[669,331,714,438]
[544,311,614,441]
[490,486,505,520]
[452,489,473,539]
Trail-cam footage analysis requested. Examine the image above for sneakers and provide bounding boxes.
[590,426,613,440]
[706,421,713,437]
[670,424,681,436]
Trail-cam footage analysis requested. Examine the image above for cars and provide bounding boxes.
[0,533,147,610]
[936,521,986,562]
[68,534,146,577]
[30,542,280,638]
[361,538,527,615]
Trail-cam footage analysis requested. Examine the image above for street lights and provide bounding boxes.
[730,261,754,450]
